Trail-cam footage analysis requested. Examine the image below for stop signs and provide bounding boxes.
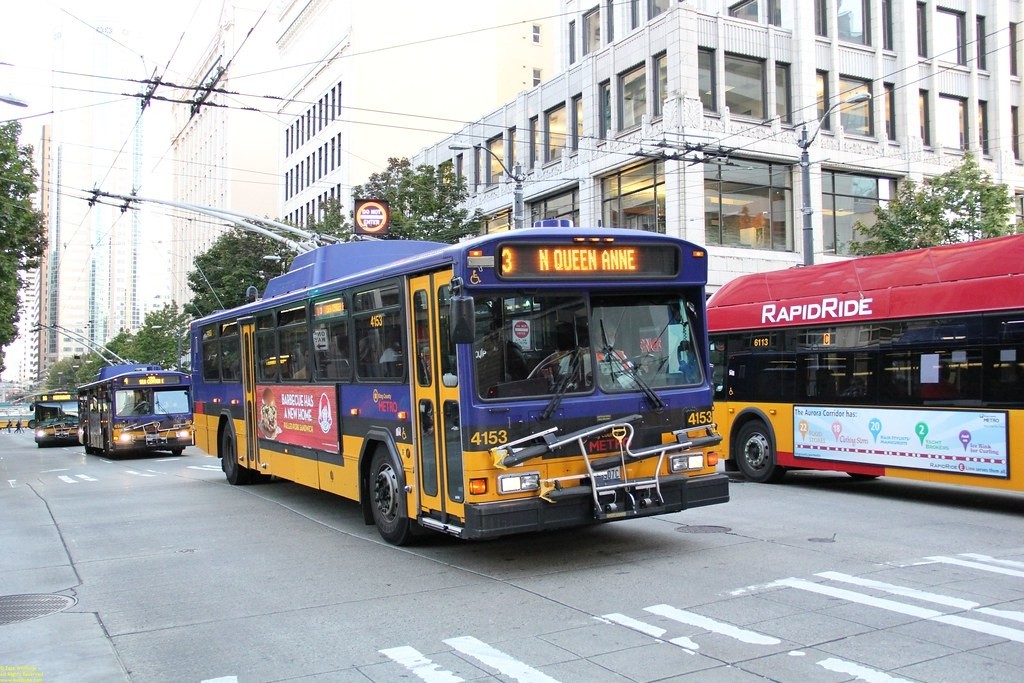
[353,198,389,236]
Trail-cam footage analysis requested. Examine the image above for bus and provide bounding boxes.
[37,322,193,463]
[0,388,62,430]
[704,232,1024,500]
[28,388,88,449]
[87,188,731,549]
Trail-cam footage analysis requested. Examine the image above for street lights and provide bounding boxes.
[151,322,183,373]
[448,142,525,228]
[796,91,872,267]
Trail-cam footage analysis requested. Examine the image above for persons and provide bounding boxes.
[7,417,25,434]
[292,333,406,381]
[593,320,635,389]
[418,346,459,437]
[479,330,528,385]
